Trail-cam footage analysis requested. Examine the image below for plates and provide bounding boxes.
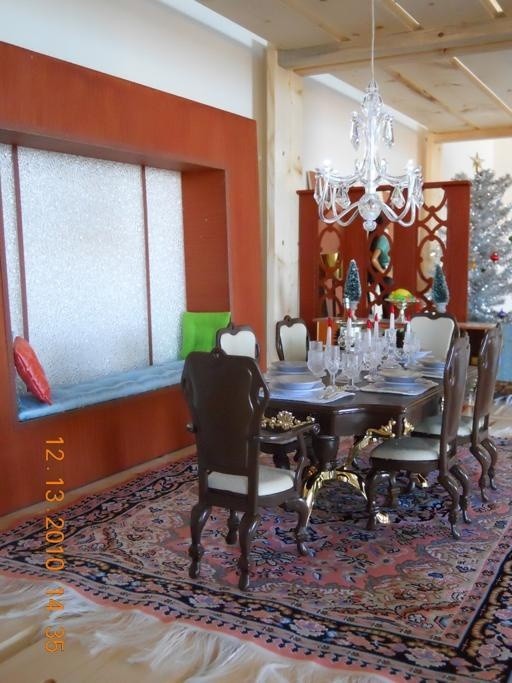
[417,367,455,373]
[268,381,325,397]
[413,349,433,359]
[268,365,309,374]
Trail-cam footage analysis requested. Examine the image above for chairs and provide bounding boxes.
[362,330,471,540]
[215,322,261,365]
[275,314,310,362]
[404,321,502,502]
[402,311,460,365]
[179,351,321,591]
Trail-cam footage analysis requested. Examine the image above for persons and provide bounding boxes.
[371,228,394,319]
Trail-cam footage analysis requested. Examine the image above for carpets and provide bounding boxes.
[1,437,512,683]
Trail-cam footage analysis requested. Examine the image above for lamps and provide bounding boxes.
[312,0,426,233]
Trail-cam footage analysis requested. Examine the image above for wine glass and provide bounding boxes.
[307,329,424,396]
[385,299,422,326]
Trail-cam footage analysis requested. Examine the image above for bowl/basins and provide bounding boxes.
[274,374,321,387]
[421,359,445,366]
[272,359,312,371]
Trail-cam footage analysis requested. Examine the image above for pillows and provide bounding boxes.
[179,312,232,361]
[12,335,54,405]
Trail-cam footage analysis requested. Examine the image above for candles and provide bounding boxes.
[346,307,352,329]
[326,320,333,346]
[390,304,394,329]
[367,319,372,346]
[373,313,378,338]
